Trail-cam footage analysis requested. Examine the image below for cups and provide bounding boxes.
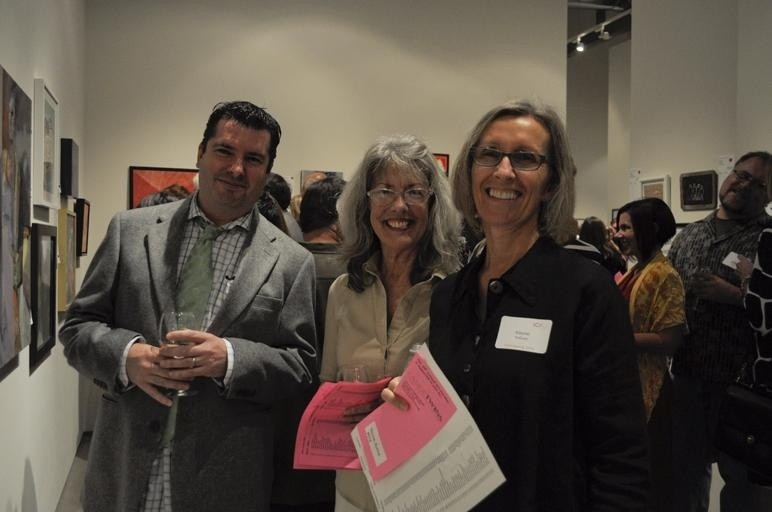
[336,366,369,383]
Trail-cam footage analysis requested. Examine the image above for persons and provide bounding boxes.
[58,100,327,512]
[579,198,688,511]
[139,185,191,209]
[260,172,349,341]
[379,96,657,511]
[668,153,770,511]
[321,136,464,512]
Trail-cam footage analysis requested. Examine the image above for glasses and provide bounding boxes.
[470,147,554,170]
[366,186,435,206]
[733,168,768,195]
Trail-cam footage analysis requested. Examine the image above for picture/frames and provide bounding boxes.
[638,170,720,212]
[31,78,90,374]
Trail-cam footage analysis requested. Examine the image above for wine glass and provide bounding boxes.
[158,311,200,398]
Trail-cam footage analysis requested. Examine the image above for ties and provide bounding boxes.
[159,225,223,450]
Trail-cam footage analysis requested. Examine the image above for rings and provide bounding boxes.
[192,356,196,367]
